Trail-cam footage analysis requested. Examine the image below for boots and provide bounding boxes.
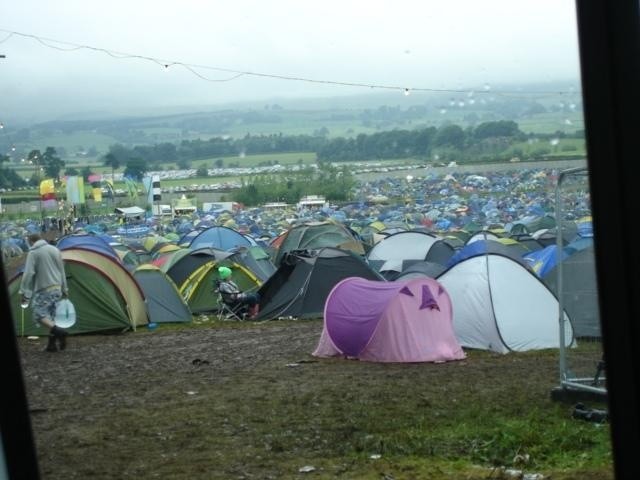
[46,335,58,352]
[50,325,69,351]
[249,304,259,321]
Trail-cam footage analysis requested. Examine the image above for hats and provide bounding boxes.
[218,266,233,280]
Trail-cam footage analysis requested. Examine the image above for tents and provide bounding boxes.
[0,152,604,364]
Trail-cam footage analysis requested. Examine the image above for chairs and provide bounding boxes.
[213,278,253,323]
